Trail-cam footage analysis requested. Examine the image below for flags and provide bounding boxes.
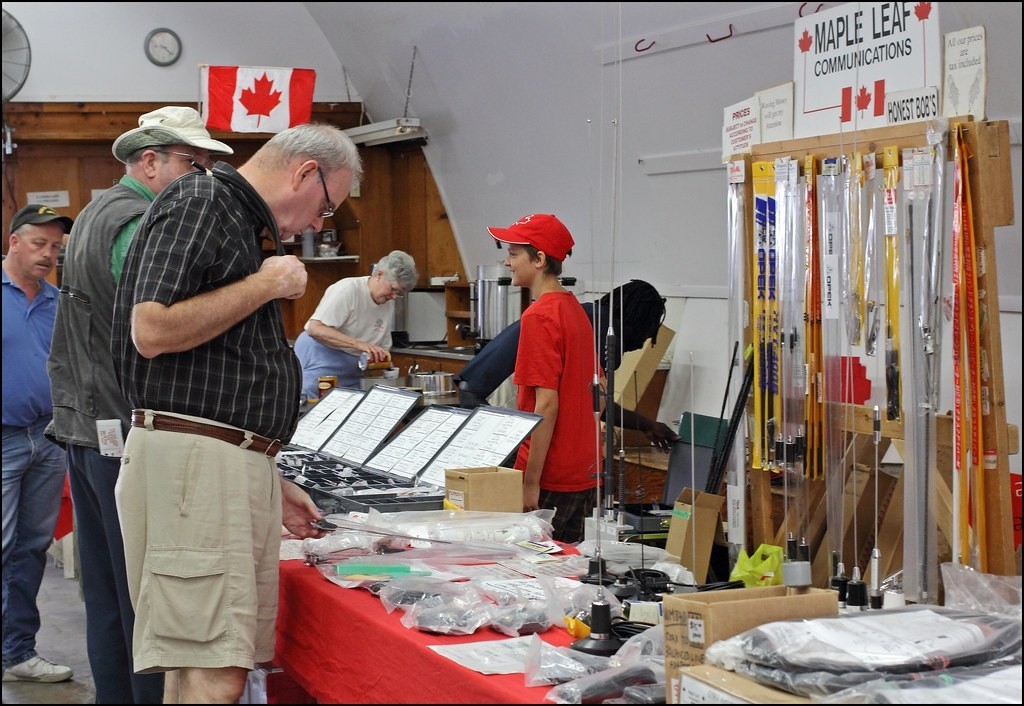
[200,65,316,134]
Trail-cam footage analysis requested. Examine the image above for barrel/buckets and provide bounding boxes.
[475,259,522,356]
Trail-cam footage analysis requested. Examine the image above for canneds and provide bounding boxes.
[358,351,391,370]
[318,376,337,399]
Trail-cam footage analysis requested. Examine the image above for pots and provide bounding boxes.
[408,370,455,394]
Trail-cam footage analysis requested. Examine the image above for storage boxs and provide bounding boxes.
[663,485,725,585]
[663,584,840,704]
[239,662,318,704]
[275,385,544,514]
[811,463,899,589]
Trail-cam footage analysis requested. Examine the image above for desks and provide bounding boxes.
[613,446,795,556]
[267,538,581,704]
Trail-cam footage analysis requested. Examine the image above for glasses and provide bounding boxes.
[388,277,405,300]
[316,165,336,219]
[149,149,214,176]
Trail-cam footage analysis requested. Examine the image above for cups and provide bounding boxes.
[383,367,400,379]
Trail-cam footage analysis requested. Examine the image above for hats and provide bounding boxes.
[112,102,234,163]
[9,203,74,236]
[486,213,575,263]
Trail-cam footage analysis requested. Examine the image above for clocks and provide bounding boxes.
[145,28,181,68]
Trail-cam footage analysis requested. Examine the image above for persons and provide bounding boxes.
[293,250,420,402]
[114,124,362,705]
[2,203,75,682]
[487,213,607,544]
[452,279,667,470]
[46,105,234,704]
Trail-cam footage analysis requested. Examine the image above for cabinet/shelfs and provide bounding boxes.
[444,280,478,347]
[391,355,467,382]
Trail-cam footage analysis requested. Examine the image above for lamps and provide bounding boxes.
[341,46,421,144]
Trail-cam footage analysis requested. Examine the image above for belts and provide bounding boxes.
[130,409,282,458]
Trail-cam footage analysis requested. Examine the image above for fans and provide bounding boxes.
[2,9,30,163]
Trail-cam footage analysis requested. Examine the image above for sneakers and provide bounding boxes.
[3,653,74,683]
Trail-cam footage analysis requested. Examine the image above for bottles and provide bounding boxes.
[318,229,338,257]
[301,228,314,257]
